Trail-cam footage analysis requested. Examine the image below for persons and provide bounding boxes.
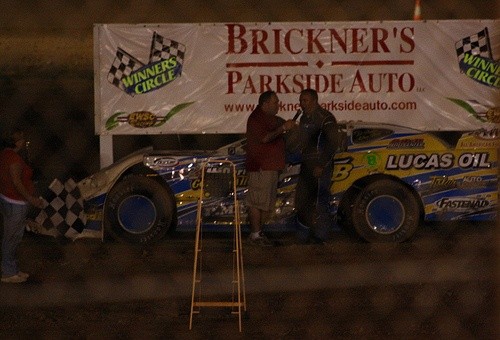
[295,88,339,246]
[0,125,45,285]
[244,89,295,248]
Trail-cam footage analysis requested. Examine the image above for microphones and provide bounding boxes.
[293,109,302,121]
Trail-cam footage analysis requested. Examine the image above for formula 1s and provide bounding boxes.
[76,121,500,245]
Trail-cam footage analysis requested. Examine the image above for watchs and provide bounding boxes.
[280,125,288,136]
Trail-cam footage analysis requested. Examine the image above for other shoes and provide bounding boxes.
[0,272,30,283]
[296,238,310,244]
[310,237,326,244]
[248,232,273,247]
